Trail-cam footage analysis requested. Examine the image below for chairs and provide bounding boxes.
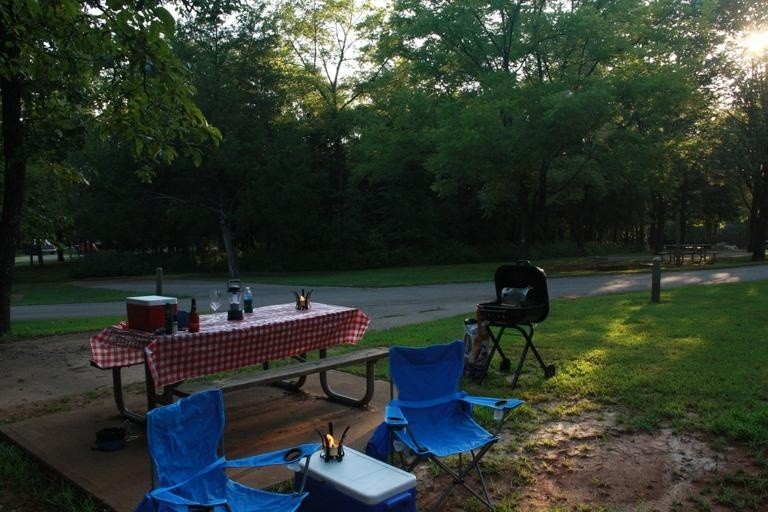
[144,383,322,511]
[362,340,524,510]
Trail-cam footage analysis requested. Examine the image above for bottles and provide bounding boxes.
[493,409,504,421]
[244,287,253,312]
[188,295,199,332]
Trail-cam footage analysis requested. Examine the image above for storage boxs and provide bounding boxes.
[126,295,177,333]
[285,444,419,512]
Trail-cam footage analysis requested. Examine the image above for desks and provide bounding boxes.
[109,299,376,427]
[662,244,715,266]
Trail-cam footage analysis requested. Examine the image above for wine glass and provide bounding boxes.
[207,288,222,322]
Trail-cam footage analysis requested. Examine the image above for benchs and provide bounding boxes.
[656,250,720,265]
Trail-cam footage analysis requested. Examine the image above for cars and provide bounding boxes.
[24,239,56,256]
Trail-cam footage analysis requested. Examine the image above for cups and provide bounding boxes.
[177,309,188,331]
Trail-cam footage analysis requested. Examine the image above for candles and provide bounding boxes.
[299,296,310,306]
